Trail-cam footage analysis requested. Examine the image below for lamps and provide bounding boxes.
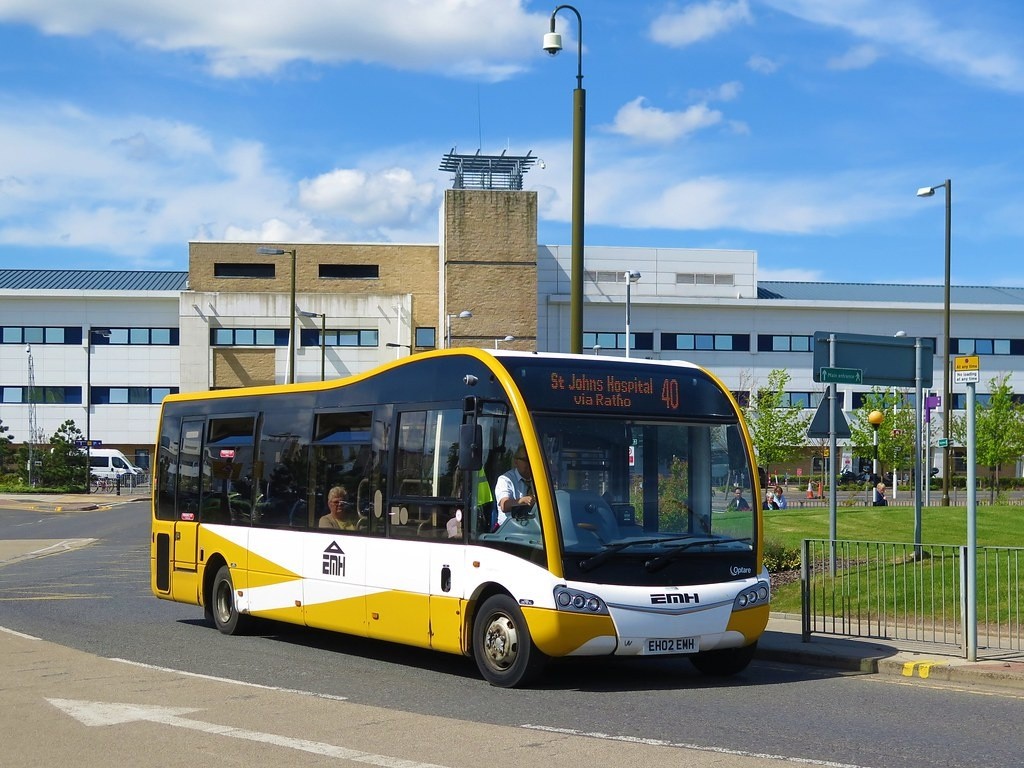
[540,163,546,169]
[26,342,30,353]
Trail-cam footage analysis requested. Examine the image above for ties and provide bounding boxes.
[526,479,533,496]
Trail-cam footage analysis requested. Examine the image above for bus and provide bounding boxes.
[152,351,772,687]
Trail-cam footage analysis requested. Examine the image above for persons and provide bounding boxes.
[784,473,788,485]
[319,487,362,531]
[873,483,888,506]
[492,446,536,533]
[762,492,779,510]
[772,486,787,510]
[731,489,749,507]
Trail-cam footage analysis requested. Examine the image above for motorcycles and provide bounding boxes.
[838,465,869,487]
[870,466,898,488]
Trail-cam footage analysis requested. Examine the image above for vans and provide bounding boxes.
[51,448,145,483]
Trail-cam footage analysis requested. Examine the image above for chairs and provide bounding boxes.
[158,447,453,544]
[491,473,503,532]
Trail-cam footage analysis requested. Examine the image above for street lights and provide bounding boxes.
[918,178,951,504]
[495,335,515,350]
[544,3,587,490]
[594,344,602,355]
[869,410,883,507]
[624,269,642,359]
[256,246,294,382]
[447,311,472,349]
[87,329,112,493]
[299,311,326,381]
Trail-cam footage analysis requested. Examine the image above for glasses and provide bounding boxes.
[516,456,528,462]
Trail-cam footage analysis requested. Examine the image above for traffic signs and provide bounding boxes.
[820,366,862,384]
[938,438,948,447]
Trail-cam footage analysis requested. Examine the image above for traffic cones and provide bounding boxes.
[805,479,816,499]
[814,481,826,499]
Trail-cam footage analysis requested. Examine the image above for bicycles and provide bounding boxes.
[88,474,115,494]
